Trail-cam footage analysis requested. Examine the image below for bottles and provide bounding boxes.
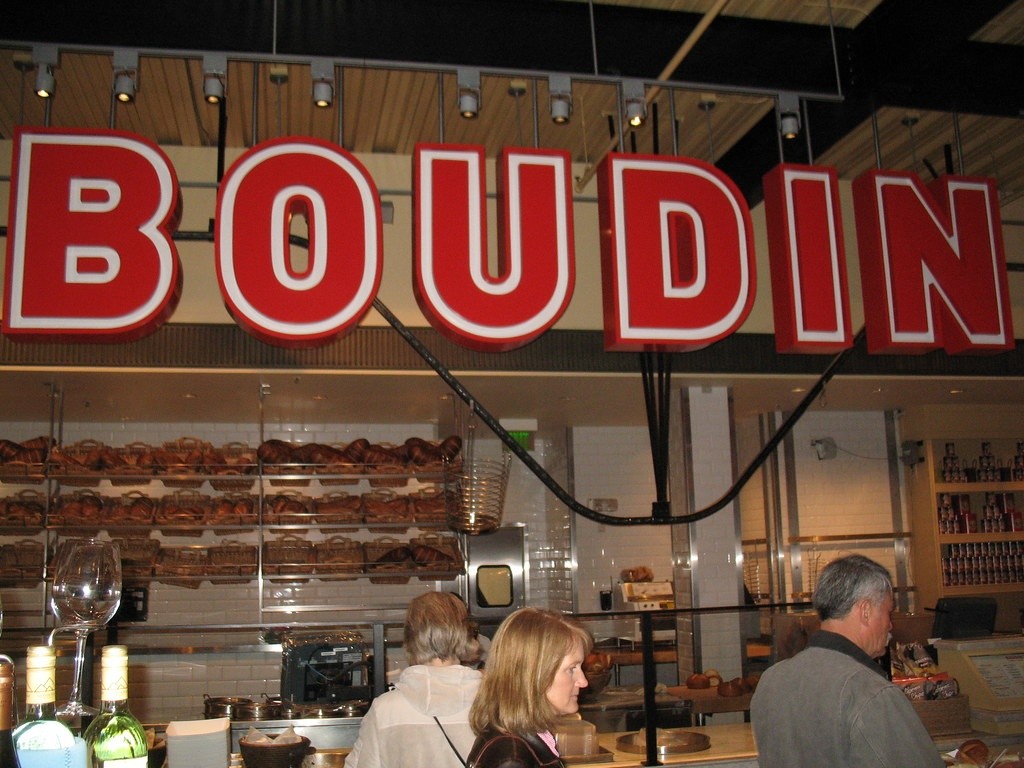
[81,645,148,767]
[0,662,21,768]
[11,646,76,768]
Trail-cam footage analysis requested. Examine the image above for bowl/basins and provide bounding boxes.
[148,738,166,768]
[202,693,369,722]
[238,734,316,768]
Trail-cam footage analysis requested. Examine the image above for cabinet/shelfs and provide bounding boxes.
[914,438,1023,618]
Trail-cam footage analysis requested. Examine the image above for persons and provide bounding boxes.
[344,590,493,768]
[750,553,947,768]
[466,608,592,768]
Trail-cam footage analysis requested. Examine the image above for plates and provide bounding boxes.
[165,718,231,768]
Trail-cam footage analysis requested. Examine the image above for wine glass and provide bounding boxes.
[50,539,122,716]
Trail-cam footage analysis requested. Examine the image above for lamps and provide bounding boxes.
[624,82,647,127]
[32,43,60,98]
[457,69,483,118]
[201,54,228,104]
[311,59,337,107]
[779,93,802,140]
[549,74,574,123]
[112,48,140,102]
